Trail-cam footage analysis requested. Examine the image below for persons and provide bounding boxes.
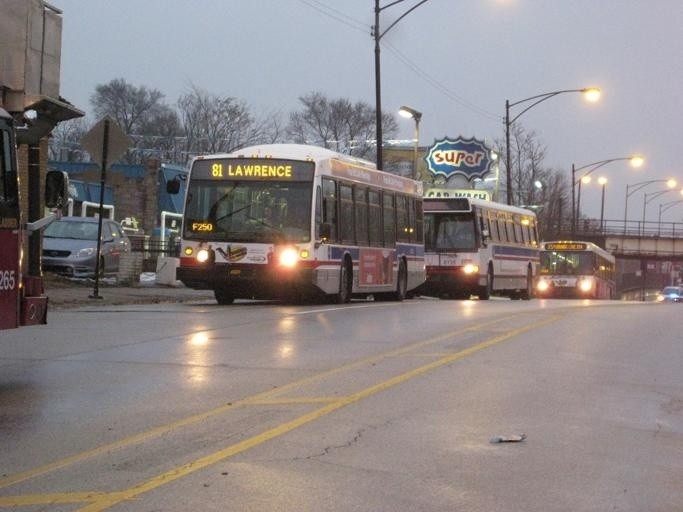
[263,206,279,224]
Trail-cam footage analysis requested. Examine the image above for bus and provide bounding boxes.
[405,196,543,301]
[533,240,617,300]
[0,106,71,333]
[164,141,428,306]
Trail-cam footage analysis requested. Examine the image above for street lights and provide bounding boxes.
[504,86,602,206]
[571,152,646,234]
[643,187,683,238]
[577,175,591,231]
[598,177,607,233]
[488,148,500,207]
[534,179,546,240]
[623,178,677,236]
[394,105,422,185]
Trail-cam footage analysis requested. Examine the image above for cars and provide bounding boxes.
[39,214,134,285]
[655,285,683,302]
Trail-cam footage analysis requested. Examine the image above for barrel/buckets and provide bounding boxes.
[155,255,180,286]
